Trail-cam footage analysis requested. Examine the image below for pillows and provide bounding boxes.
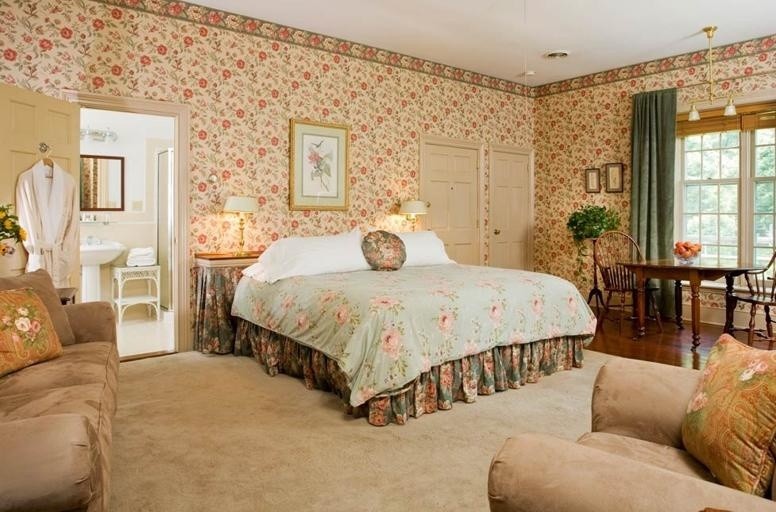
[363,230,407,271]
[0,271,78,378]
[681,333,776,500]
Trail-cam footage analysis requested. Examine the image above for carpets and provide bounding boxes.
[116,350,624,511]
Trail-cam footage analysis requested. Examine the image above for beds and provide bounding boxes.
[236,227,601,426]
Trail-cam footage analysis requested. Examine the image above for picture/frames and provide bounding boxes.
[584,163,622,193]
[289,118,349,212]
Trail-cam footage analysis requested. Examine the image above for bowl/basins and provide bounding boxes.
[672,253,699,264]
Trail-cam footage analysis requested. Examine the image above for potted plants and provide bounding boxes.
[566,204,622,291]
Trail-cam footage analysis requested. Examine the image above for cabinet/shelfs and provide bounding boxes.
[112,266,160,325]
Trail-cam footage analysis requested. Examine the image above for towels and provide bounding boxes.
[127,247,157,266]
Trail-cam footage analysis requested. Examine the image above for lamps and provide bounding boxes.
[223,196,261,258]
[685,26,738,122]
[399,199,429,232]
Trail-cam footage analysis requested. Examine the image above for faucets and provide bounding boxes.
[85,235,94,246]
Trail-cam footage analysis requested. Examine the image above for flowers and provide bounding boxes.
[0,203,27,244]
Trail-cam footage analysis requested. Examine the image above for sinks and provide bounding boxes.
[80,240,125,264]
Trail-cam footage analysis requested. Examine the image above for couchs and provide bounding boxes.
[0,301,119,511]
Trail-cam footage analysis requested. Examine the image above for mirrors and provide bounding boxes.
[80,154,125,211]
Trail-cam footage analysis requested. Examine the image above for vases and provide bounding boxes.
[1,239,27,279]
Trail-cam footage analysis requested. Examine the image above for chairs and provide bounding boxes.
[487,357,776,512]
[595,230,775,346]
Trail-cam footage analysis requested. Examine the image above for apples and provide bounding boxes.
[671,242,702,258]
